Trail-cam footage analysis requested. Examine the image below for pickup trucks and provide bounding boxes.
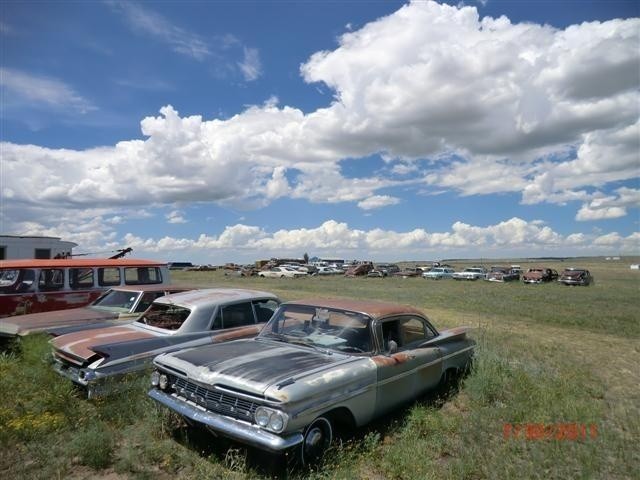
[452,267,483,281]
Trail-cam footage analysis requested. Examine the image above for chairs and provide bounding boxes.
[218,306,274,327]
[304,315,402,352]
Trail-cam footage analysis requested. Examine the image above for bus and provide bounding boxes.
[0,259,171,319]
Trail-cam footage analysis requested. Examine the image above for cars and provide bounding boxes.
[0,286,196,354]
[167,259,399,279]
[396,267,423,278]
[485,264,522,283]
[419,265,454,281]
[147,297,478,478]
[558,267,594,286]
[46,287,283,397]
[523,268,557,284]
